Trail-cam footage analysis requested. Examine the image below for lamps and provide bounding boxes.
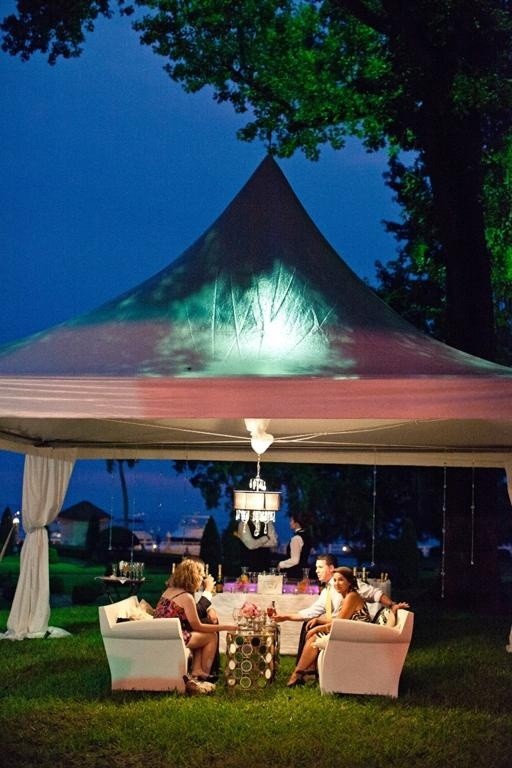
[234,435,280,536]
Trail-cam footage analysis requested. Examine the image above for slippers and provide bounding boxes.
[198,674,218,683]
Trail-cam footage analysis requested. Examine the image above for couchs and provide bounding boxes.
[98,596,191,695]
[309,601,414,700]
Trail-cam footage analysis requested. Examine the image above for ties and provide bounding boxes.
[324,584,332,621]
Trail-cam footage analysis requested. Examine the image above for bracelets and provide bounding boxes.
[326,624,330,634]
[388,601,397,607]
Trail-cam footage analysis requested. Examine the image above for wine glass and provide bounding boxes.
[252,572,258,593]
[233,608,242,626]
[267,608,273,626]
[279,572,288,593]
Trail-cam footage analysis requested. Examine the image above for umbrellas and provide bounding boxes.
[0,151,512,640]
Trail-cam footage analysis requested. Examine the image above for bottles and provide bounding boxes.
[216,563,226,592]
[204,564,211,575]
[169,562,179,574]
[379,571,388,588]
[352,567,359,579]
[361,567,369,585]
[110,559,146,579]
[268,602,279,623]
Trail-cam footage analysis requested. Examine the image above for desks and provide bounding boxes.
[193,593,322,656]
[95,576,152,605]
[227,622,280,691]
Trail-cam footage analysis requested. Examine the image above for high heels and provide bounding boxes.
[281,679,303,689]
[295,669,319,683]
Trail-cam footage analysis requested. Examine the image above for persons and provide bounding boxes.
[286,567,372,687]
[276,510,316,580]
[237,514,279,573]
[173,555,218,674]
[153,559,239,681]
[272,553,411,667]
